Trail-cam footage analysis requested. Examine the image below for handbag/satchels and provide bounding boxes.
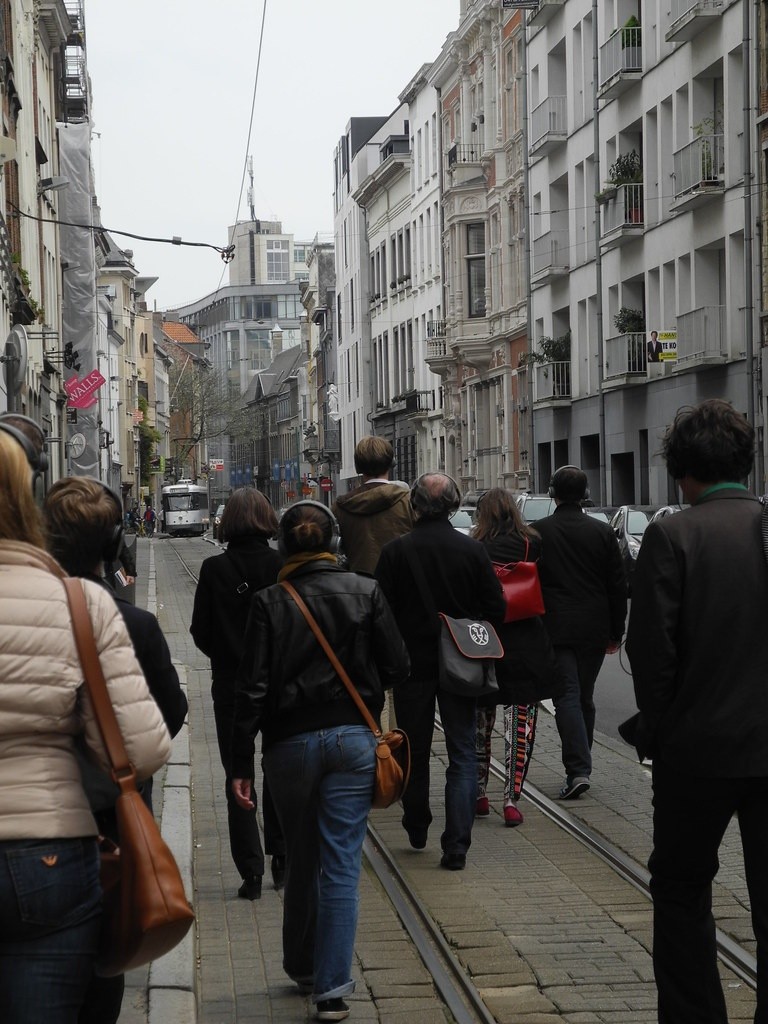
[60,569,193,976]
[479,531,545,622]
[434,613,504,698]
[372,727,412,809]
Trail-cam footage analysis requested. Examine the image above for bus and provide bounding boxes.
[159,436,212,538]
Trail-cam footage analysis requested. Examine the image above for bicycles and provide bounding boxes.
[123,512,159,539]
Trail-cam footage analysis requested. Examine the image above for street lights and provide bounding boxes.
[208,477,215,507]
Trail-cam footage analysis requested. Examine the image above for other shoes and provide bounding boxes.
[504,806,523,826]
[270,857,285,889]
[440,850,466,869]
[236,878,262,900]
[474,796,490,818]
[297,977,312,990]
[315,998,351,1020]
[401,817,426,849]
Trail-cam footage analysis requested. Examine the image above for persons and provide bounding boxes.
[192,486,287,900]
[465,486,550,828]
[334,436,422,732]
[374,472,507,868]
[646,330,663,362]
[126,505,164,538]
[530,465,629,799]
[617,400,768,1024]
[228,500,412,1020]
[0,414,190,1024]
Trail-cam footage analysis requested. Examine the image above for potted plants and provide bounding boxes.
[690,100,724,186]
[610,14,642,70]
[594,188,616,204]
[519,331,570,395]
[609,148,644,224]
[613,307,646,371]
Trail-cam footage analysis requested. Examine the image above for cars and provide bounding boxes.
[446,492,691,600]
[210,504,226,539]
[270,506,291,541]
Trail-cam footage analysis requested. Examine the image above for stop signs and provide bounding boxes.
[320,479,333,492]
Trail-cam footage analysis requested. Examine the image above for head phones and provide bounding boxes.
[83,479,125,562]
[549,465,590,500]
[410,471,461,511]
[476,492,487,517]
[0,414,49,495]
[276,499,342,555]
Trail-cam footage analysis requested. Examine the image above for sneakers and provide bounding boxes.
[559,775,590,799]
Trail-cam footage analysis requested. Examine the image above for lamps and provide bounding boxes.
[46,342,81,372]
[97,350,106,357]
[111,376,121,381]
[37,175,69,195]
[117,401,122,406]
